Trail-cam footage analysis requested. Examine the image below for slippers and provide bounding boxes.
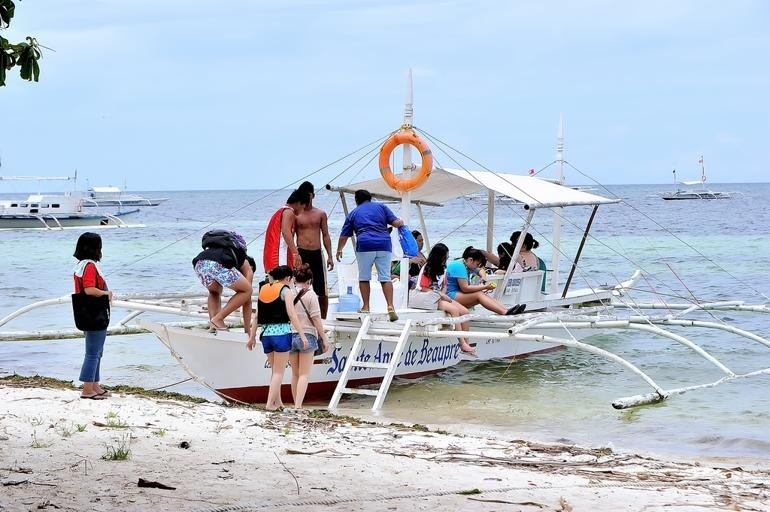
[208,321,227,332]
[358,308,370,313]
[81,391,111,400]
[388,306,398,321]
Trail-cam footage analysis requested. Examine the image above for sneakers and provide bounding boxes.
[505,304,525,314]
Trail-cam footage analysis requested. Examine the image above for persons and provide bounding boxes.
[296,180,334,319]
[336,189,404,322]
[287,267,331,412]
[191,246,257,337]
[244,265,309,410]
[388,228,548,354]
[72,231,113,399]
[263,189,311,280]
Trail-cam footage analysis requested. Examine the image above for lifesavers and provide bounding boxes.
[380,132,432,191]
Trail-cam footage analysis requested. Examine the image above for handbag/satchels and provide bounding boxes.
[409,289,441,310]
[71,292,110,331]
[201,231,246,250]
[399,225,419,256]
[313,333,326,355]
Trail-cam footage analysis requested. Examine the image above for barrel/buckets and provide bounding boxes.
[335,286,360,312]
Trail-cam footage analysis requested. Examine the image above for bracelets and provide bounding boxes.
[337,251,342,253]
[327,256,333,258]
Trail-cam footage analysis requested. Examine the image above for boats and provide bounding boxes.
[465,181,600,203]
[0,61,770,409]
[82,186,167,211]
[660,153,738,199]
[0,173,140,230]
